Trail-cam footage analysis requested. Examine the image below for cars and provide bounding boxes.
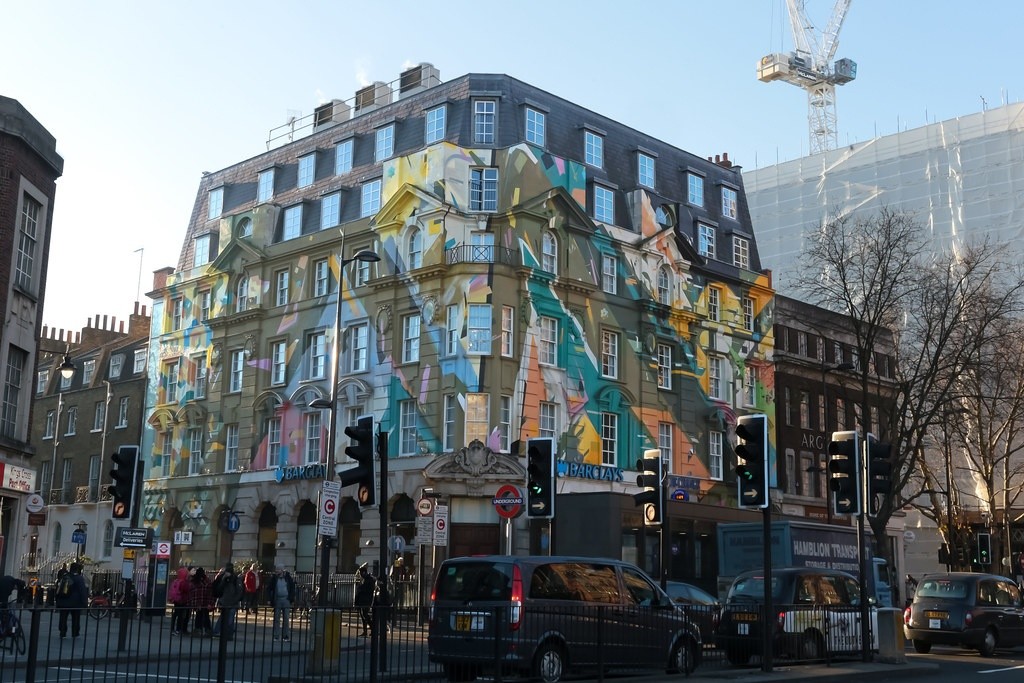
[652,579,723,645]
[902,572,1024,657]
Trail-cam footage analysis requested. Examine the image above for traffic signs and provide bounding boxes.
[113,526,154,549]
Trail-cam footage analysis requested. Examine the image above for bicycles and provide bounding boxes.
[0,598,27,655]
[88,587,142,620]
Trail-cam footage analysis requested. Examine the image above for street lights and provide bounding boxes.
[306,223,383,608]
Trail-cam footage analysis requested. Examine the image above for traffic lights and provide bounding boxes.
[866,432,893,518]
[526,437,555,519]
[977,532,992,565]
[828,432,861,516]
[735,414,769,510]
[107,445,139,520]
[957,547,967,564]
[635,449,662,525]
[344,414,377,508]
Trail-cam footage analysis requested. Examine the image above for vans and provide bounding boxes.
[427,555,703,683]
[718,567,886,668]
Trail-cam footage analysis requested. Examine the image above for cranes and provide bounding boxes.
[756,0,858,155]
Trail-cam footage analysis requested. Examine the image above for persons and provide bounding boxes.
[390,557,407,610]
[238,562,262,615]
[57,563,68,578]
[906,574,916,598]
[266,562,296,641]
[169,563,239,640]
[0,575,26,601]
[354,562,372,636]
[54,563,82,639]
[372,577,392,632]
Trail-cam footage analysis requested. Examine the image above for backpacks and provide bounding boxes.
[55,572,77,597]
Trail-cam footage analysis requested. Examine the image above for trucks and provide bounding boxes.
[716,518,898,615]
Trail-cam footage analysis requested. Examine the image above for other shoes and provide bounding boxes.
[72,632,80,638]
[60,633,67,639]
